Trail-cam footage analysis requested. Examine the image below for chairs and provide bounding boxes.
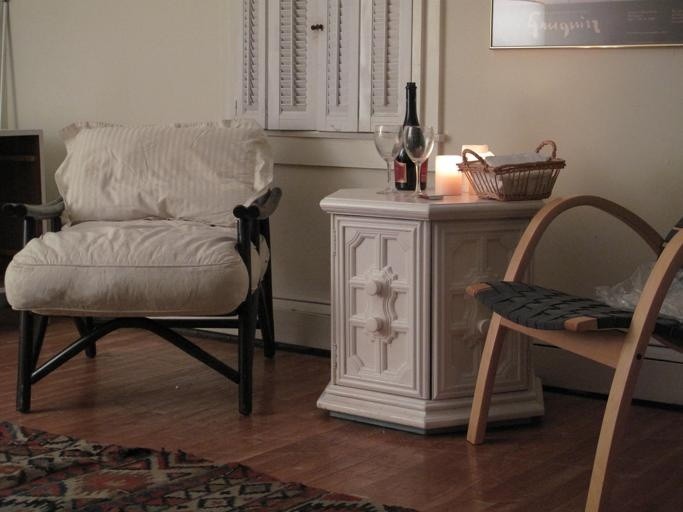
[0,118,281,419]
[463,197,682,512]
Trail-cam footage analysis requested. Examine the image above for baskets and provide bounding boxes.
[457,139,565,200]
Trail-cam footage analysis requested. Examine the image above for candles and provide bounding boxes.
[435,154,463,195]
[461,144,489,196]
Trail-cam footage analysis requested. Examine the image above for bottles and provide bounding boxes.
[393,82,428,190]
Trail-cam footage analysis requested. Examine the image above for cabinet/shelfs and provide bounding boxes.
[315,183,543,437]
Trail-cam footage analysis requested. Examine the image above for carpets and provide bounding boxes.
[0,418,420,512]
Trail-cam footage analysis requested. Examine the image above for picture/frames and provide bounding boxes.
[488,0,681,52]
[0,127,48,294]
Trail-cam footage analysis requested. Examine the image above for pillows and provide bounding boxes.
[56,120,270,225]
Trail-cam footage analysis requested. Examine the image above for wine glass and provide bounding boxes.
[374,124,406,197]
[403,125,436,201]
